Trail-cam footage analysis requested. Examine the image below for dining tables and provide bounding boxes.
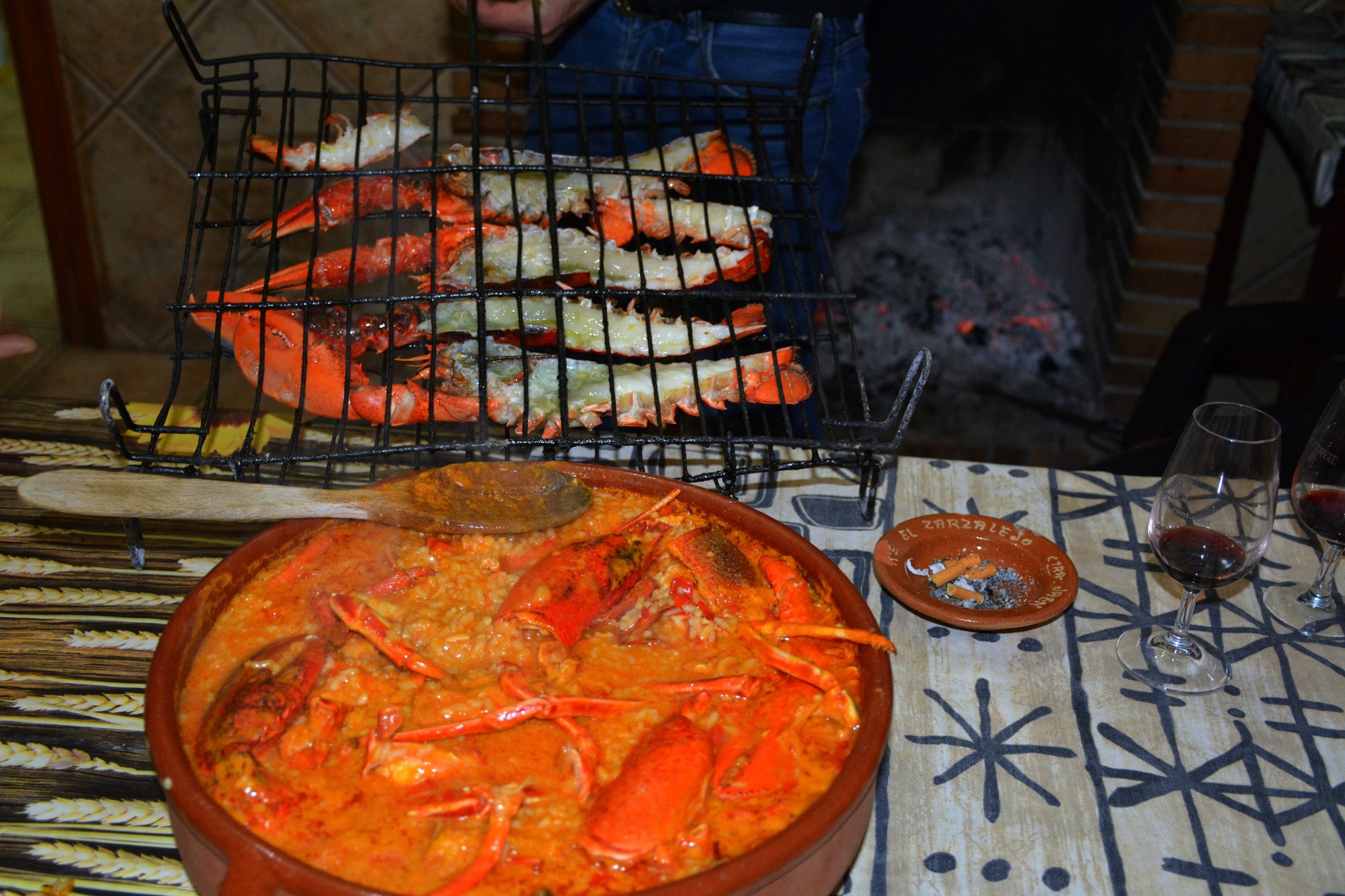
[0,405,1345,894]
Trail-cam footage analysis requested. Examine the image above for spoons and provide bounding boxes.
[14,457,594,536]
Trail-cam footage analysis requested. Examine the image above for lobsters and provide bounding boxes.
[189,290,769,361]
[233,310,811,441]
[236,196,775,291]
[245,127,758,246]
[198,488,900,896]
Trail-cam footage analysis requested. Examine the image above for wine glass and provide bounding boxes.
[1116,402,1281,694]
[1261,376,1345,637]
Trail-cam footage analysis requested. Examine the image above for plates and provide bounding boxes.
[873,513,1079,628]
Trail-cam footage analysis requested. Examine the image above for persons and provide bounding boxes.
[455,0,874,447]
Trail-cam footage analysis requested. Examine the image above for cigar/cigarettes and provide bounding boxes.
[920,547,1025,613]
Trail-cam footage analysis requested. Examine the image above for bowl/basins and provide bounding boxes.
[143,463,895,896]
